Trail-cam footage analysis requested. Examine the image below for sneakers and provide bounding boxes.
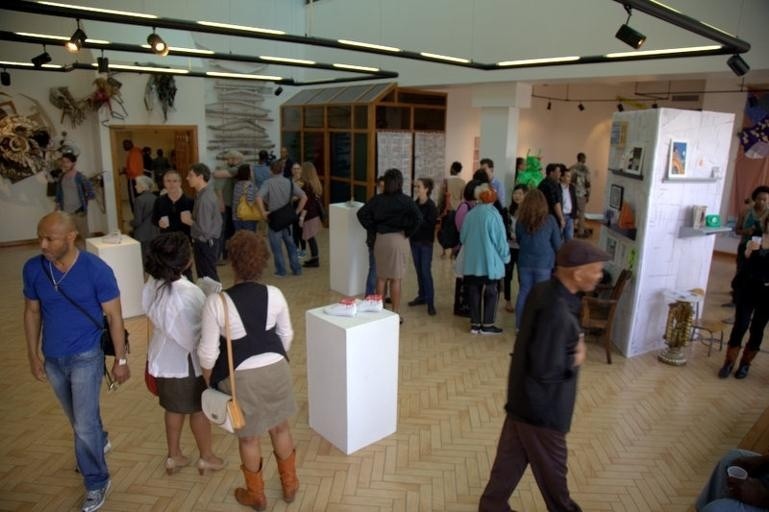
[274,250,319,276]
[408,300,513,334]
[76,439,111,472]
[324,299,356,316]
[356,295,383,311]
[80,480,112,512]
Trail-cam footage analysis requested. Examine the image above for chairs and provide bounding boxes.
[581,269,632,364]
[689,289,727,357]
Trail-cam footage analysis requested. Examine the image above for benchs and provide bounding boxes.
[737,406,769,455]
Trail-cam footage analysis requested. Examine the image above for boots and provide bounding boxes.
[723,341,741,377]
[736,344,760,378]
[235,457,266,510]
[273,448,299,502]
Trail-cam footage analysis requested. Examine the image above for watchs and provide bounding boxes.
[113,357,127,367]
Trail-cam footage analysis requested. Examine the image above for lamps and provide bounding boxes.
[726,54,750,77]
[65,18,87,52]
[31,45,51,65]
[147,27,166,54]
[617,8,647,50]
[0,67,10,86]
[546,102,662,112]
[275,85,283,96]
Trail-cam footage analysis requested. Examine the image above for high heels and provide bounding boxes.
[165,456,191,474]
[197,457,229,475]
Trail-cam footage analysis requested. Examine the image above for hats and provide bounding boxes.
[557,240,609,266]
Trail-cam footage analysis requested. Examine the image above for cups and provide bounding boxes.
[161,215,170,227]
[181,210,190,222]
[111,228,121,241]
[727,466,747,491]
[752,236,761,250]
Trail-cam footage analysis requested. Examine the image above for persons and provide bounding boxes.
[357,168,423,324]
[407,178,438,315]
[505,152,591,332]
[455,158,511,335]
[716,183,769,381]
[196,227,300,510]
[438,162,466,257]
[118,139,322,285]
[479,236,611,512]
[22,209,130,512]
[140,230,227,476]
[51,152,105,240]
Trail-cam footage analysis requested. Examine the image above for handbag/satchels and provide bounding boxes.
[145,361,159,396]
[440,192,452,216]
[454,277,472,317]
[438,211,459,248]
[268,203,298,231]
[451,246,464,273]
[201,388,246,434]
[100,316,129,355]
[317,200,328,227]
[236,193,262,221]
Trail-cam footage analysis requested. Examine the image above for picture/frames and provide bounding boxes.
[667,139,689,179]
[603,230,620,266]
[623,143,645,176]
[609,184,624,211]
